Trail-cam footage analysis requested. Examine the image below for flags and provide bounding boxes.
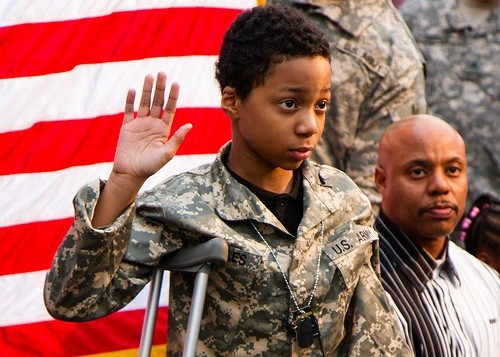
[1,0,266,357]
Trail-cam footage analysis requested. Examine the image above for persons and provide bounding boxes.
[42,0,417,357]
[394,1,500,252]
[283,0,429,228]
[369,114,500,357]
[459,192,500,277]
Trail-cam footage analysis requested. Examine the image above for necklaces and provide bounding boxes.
[248,219,325,349]
